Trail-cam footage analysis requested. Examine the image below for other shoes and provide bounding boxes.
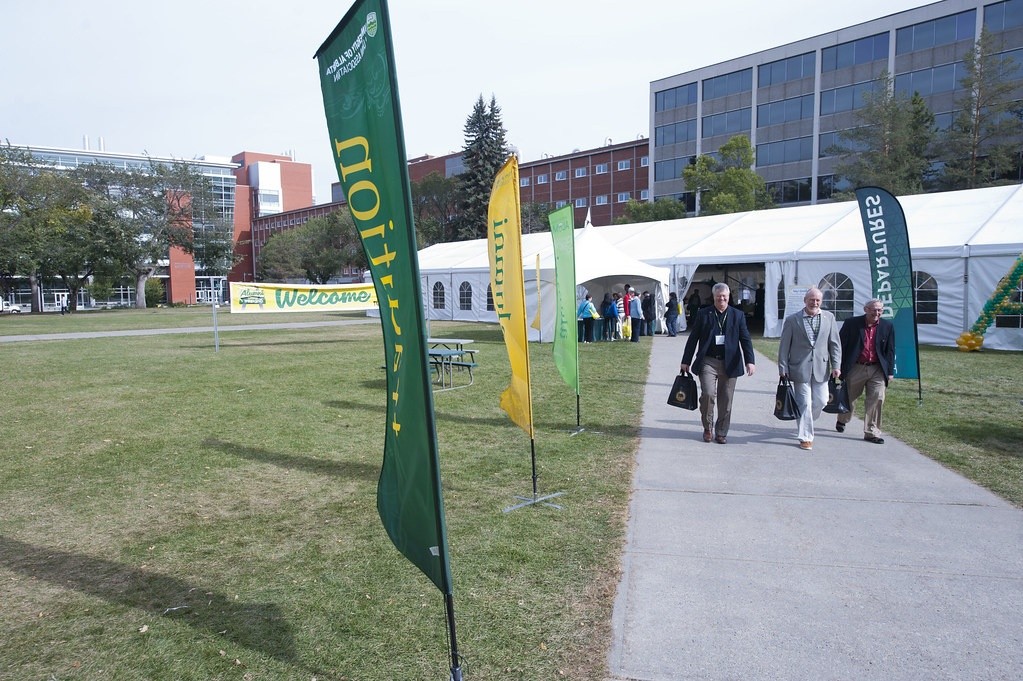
[837,419,845,432]
[611,336,616,341]
[587,342,592,344]
[801,439,812,450]
[864,436,884,444]
[584,341,586,344]
[666,334,675,337]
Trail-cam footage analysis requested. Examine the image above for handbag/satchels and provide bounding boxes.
[822,373,850,413]
[590,308,600,321]
[668,370,698,410]
[664,311,670,317]
[675,302,681,316]
[623,318,630,339]
[774,374,800,420]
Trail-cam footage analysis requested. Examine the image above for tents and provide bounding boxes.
[366,183,1023,352]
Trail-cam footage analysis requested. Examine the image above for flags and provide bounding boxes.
[531,254,540,331]
[548,206,578,394]
[316,0,453,594]
[855,187,921,379]
[487,160,534,440]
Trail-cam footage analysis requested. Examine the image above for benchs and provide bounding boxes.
[381,349,480,384]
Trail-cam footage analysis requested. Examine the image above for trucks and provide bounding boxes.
[0,295,21,314]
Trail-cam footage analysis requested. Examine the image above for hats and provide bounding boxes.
[628,286,634,293]
[642,290,649,295]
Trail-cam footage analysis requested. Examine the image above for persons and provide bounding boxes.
[600,284,645,343]
[835,298,895,444]
[577,294,596,344]
[680,282,755,444]
[754,283,765,303]
[778,288,842,450]
[642,290,656,336]
[664,292,678,337]
[688,289,701,325]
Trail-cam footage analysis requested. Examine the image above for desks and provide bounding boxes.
[428,336,475,371]
[428,348,466,387]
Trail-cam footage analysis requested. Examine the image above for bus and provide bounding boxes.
[0,274,95,312]
[141,275,227,306]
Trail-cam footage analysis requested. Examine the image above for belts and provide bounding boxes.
[855,361,880,366]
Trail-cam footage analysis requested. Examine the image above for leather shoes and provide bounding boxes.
[703,425,712,442]
[716,434,726,445]
